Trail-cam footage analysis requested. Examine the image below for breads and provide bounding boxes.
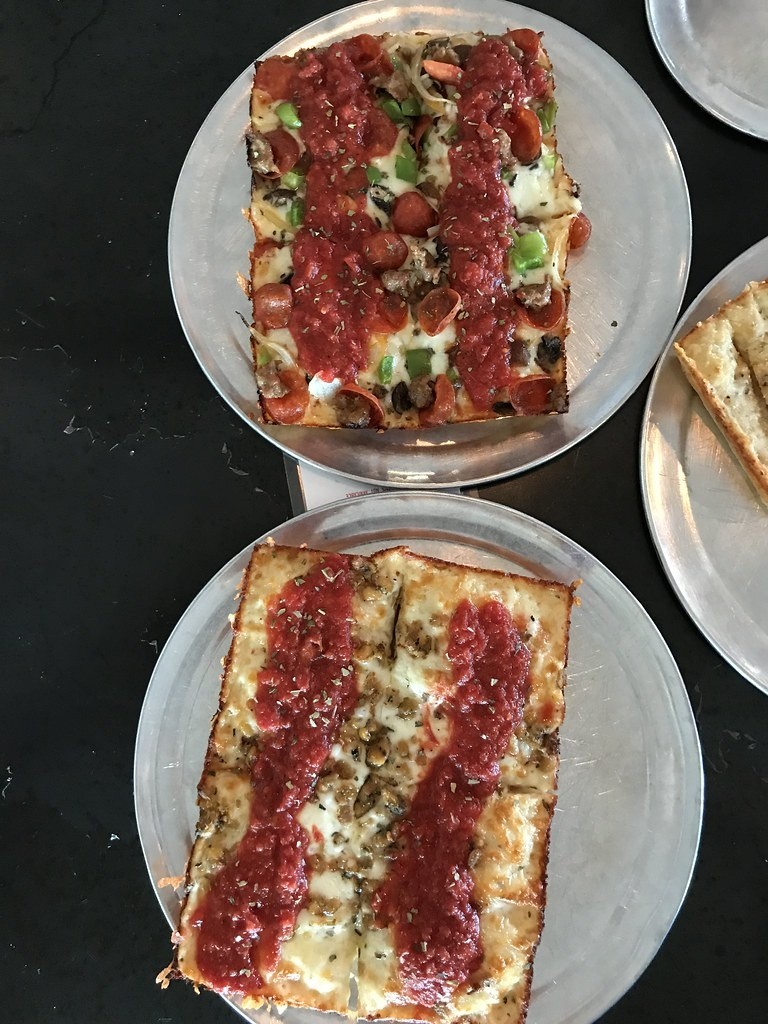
[673,280,768,506]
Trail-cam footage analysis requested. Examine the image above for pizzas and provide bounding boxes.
[173,544,578,1024]
[249,29,583,425]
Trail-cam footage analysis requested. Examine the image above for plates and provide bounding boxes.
[167,1,693,488]
[640,233,768,693]
[646,0,768,141]
[134,490,705,1024]
